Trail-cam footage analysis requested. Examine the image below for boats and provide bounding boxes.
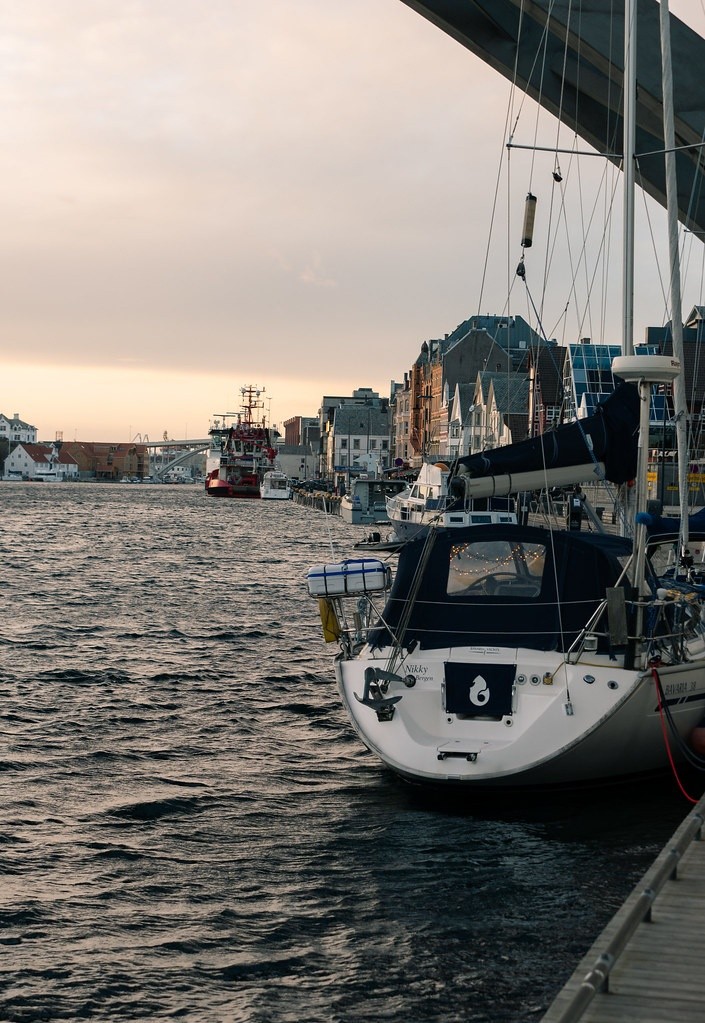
[260,469,289,500]
[383,463,509,553]
[2,472,70,484]
[117,470,203,486]
[202,386,284,498]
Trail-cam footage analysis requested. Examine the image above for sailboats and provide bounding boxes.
[306,0,705,798]
